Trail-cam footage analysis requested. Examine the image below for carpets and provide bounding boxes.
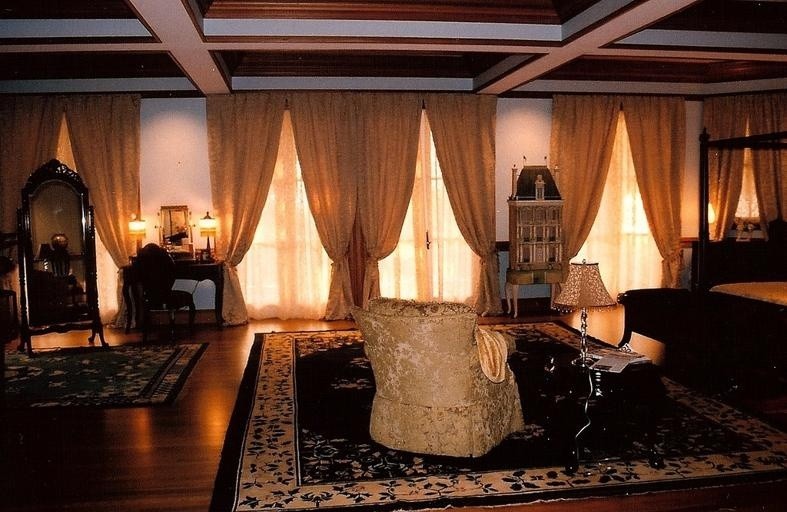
[208,319,785,512]
[0,340,210,414]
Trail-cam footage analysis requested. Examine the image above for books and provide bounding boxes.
[589,340,651,363]
[588,350,635,376]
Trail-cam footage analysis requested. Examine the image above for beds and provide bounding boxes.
[690,120,785,395]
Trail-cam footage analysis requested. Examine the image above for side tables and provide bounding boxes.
[512,344,666,474]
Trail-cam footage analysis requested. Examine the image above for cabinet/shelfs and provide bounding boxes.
[502,157,572,322]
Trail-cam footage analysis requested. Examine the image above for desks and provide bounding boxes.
[119,259,227,333]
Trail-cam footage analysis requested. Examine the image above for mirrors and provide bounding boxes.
[159,204,191,253]
[13,156,111,355]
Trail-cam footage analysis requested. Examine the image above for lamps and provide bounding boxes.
[198,212,219,263]
[34,243,55,263]
[551,259,618,364]
[128,213,147,255]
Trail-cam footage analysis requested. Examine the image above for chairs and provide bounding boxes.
[344,303,518,467]
[132,243,194,343]
